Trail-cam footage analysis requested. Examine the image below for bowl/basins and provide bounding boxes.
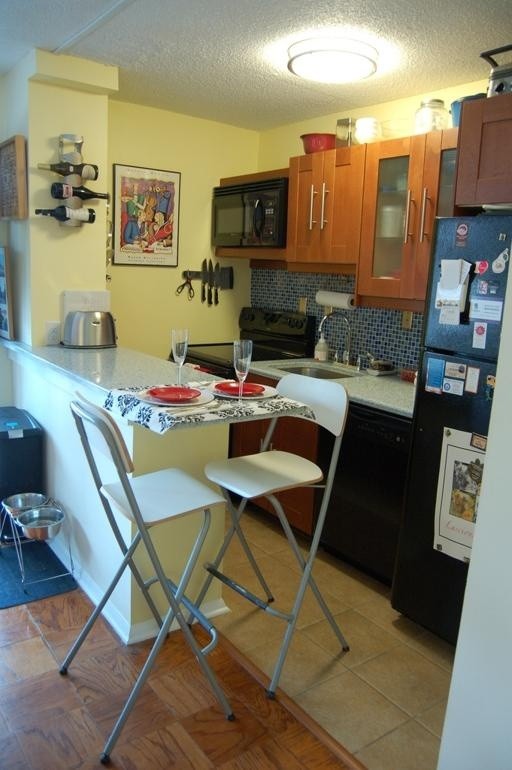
[15,508,64,539]
[300,133,337,153]
[3,492,50,518]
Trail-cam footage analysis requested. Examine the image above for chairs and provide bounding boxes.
[206,373,350,700]
[60,390,237,766]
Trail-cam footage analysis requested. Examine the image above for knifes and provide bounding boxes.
[208,259,214,305]
[215,263,220,305]
[201,259,207,302]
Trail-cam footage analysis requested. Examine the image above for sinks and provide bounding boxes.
[271,365,363,379]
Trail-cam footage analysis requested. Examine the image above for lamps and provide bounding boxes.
[286,34,381,86]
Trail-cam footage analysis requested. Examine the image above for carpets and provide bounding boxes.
[1,539,78,610]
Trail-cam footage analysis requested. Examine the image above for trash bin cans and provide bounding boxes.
[0,406,43,547]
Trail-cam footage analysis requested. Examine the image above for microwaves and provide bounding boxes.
[211,178,288,247]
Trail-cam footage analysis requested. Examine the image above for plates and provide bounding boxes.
[138,386,212,407]
[208,381,280,399]
[149,386,202,402]
[215,382,264,395]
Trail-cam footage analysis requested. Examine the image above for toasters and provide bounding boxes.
[63,311,118,347]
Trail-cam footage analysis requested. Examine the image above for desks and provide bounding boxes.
[0,344,315,645]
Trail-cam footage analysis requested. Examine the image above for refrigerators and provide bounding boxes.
[391,212,512,646]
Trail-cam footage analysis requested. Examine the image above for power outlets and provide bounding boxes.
[401,311,415,331]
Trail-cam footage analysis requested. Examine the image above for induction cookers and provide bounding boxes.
[180,308,315,363]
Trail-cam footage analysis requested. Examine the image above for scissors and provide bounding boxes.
[177,268,196,297]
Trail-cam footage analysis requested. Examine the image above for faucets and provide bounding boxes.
[318,312,352,363]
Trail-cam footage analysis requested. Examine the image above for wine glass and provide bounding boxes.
[171,327,188,387]
[234,340,253,409]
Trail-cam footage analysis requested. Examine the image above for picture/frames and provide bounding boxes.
[112,163,181,270]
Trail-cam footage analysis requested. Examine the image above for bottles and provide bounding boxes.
[35,206,96,223]
[36,161,99,180]
[51,184,111,200]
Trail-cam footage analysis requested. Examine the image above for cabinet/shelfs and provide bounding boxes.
[168,353,410,590]
[455,92,512,207]
